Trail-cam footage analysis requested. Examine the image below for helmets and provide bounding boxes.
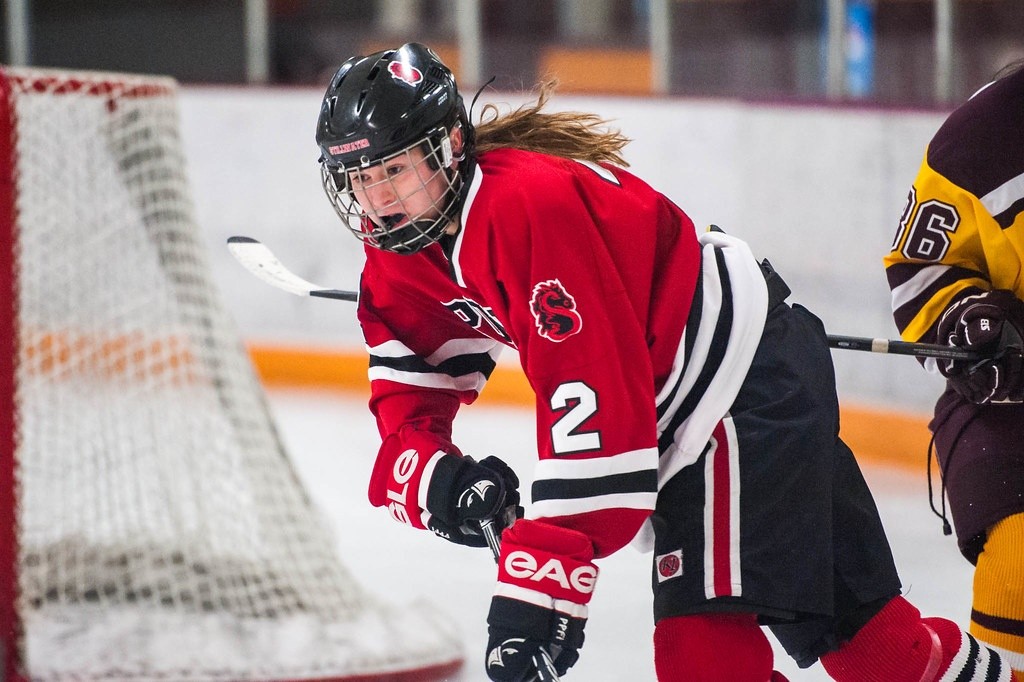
[316,42,476,254]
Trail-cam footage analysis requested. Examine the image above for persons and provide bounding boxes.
[316,41,1012,682]
[882,63,1024,682]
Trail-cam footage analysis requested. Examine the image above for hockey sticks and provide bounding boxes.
[477,517,562,681]
[225,236,1024,361]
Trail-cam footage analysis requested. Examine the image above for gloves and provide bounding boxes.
[937,288,1024,406]
[369,428,525,547]
[485,519,599,682]
